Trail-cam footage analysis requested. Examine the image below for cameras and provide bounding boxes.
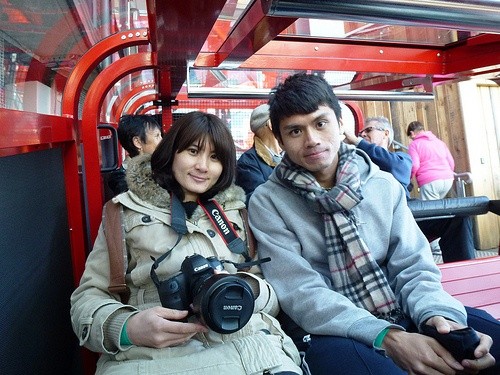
[155,254,255,334]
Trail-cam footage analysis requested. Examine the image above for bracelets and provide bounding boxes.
[120,320,133,346]
[375,328,390,348]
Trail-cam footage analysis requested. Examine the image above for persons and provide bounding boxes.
[247,72,500,375]
[106,101,476,263]
[70,112,301,375]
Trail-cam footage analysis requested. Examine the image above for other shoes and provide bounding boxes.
[431,247,441,255]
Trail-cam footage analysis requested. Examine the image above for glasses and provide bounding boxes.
[357,126,384,137]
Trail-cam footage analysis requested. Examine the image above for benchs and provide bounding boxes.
[437,255,500,323]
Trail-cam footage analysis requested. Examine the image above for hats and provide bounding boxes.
[250,104,270,131]
